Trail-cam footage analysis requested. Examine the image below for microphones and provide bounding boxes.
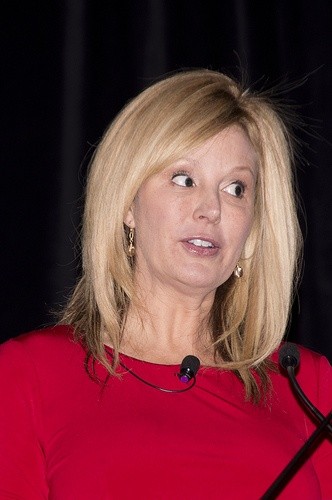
[279,342,332,434]
[179,355,200,382]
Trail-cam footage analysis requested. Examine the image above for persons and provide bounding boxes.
[0,72,332,500]
